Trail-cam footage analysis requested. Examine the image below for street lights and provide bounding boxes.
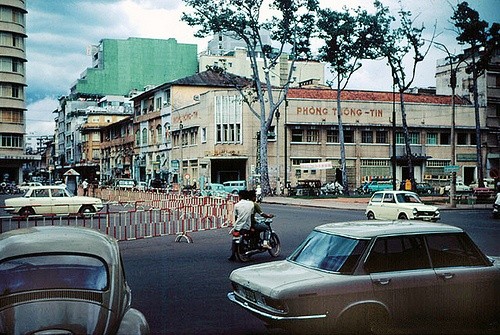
[275,79,294,196]
[449,46,485,208]
[163,102,184,196]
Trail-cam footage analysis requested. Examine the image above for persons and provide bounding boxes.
[493,192,500,209]
[83,177,107,196]
[227,186,274,261]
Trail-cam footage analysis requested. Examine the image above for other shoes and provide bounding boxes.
[228,256,237,260]
[263,244,272,250]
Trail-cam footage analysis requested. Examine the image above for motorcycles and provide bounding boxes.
[232,213,281,263]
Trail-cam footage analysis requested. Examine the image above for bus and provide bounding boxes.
[277,179,321,197]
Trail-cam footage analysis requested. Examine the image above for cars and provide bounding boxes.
[0,226,150,335]
[434,177,499,198]
[319,181,345,196]
[225,220,500,335]
[364,190,441,225]
[4,186,104,220]
[359,178,401,193]
[130,182,147,193]
[16,182,43,195]
[399,182,434,196]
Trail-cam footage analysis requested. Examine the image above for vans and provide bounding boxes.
[200,181,247,198]
